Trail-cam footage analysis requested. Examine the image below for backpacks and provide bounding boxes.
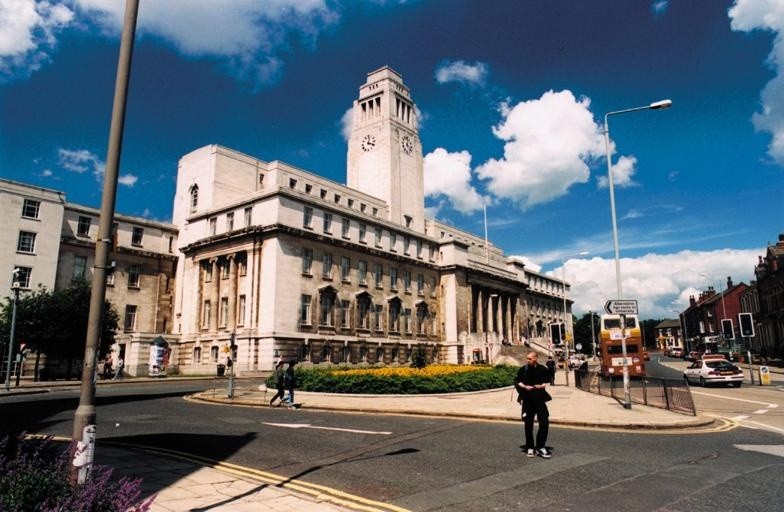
[512,364,528,394]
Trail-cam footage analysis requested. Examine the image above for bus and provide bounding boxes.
[600,314,647,378]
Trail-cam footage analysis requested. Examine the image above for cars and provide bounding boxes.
[683,357,744,389]
[559,355,580,368]
[644,352,649,361]
[668,347,685,358]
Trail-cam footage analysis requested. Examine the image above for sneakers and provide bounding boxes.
[526,447,551,458]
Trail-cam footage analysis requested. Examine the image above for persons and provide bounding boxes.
[222,356,236,377]
[80,354,124,405]
[515,351,553,458]
[162,351,170,376]
[278,360,297,409]
[545,340,588,388]
[270,361,285,406]
[501,336,530,347]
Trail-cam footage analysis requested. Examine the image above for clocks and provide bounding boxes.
[402,135,413,154]
[361,135,375,151]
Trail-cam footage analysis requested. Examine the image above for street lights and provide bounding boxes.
[484,293,499,366]
[603,99,673,406]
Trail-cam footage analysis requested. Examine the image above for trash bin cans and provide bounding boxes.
[574,370,589,387]
[217,365,226,377]
